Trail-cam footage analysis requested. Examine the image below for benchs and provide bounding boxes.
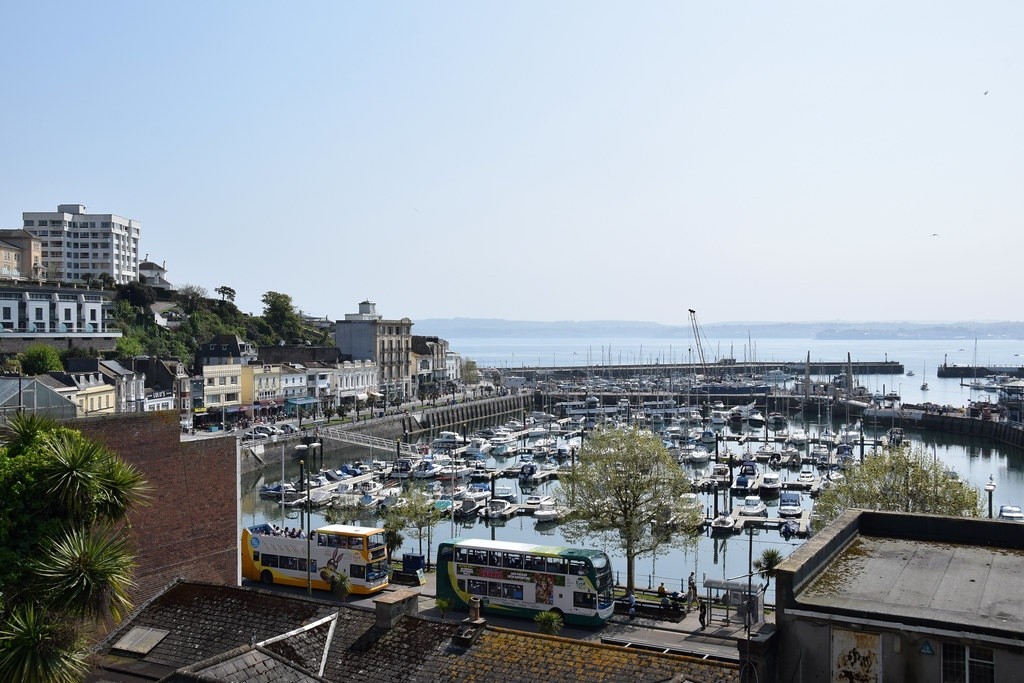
[613,585,677,602]
[613,598,684,613]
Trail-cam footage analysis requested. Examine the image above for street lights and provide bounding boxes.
[295,442,321,596]
[984,481,996,519]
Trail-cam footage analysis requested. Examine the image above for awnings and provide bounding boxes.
[357,391,384,400]
[193,396,322,417]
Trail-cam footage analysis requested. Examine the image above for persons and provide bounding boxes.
[262,555,277,567]
[456,550,588,575]
[299,558,316,572]
[258,530,265,536]
[658,572,753,634]
[503,584,522,600]
[280,556,297,569]
[197,409,322,435]
[489,583,501,597]
[328,535,363,548]
[310,530,316,540]
[586,594,592,604]
[468,581,486,595]
[272,524,304,539]
[629,591,635,619]
[318,533,326,546]
[351,566,364,575]
[592,597,596,609]
[352,408,405,422]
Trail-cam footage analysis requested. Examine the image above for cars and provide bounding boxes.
[375,400,391,408]
[244,424,301,439]
[537,380,548,388]
[557,384,570,389]
[691,389,708,394]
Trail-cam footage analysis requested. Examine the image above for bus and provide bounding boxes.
[242,523,389,595]
[437,538,615,628]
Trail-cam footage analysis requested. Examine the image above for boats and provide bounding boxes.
[258,369,1024,534]
